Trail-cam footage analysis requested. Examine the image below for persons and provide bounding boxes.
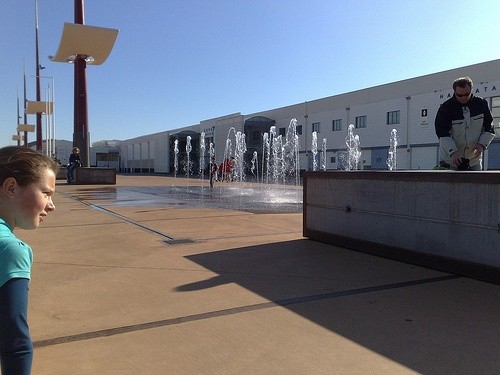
[0,146,60,375]
[208,157,219,189]
[67,147,84,184]
[218,155,238,182]
[433,76,496,171]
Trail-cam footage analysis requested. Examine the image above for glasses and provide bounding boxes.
[455,90,471,97]
